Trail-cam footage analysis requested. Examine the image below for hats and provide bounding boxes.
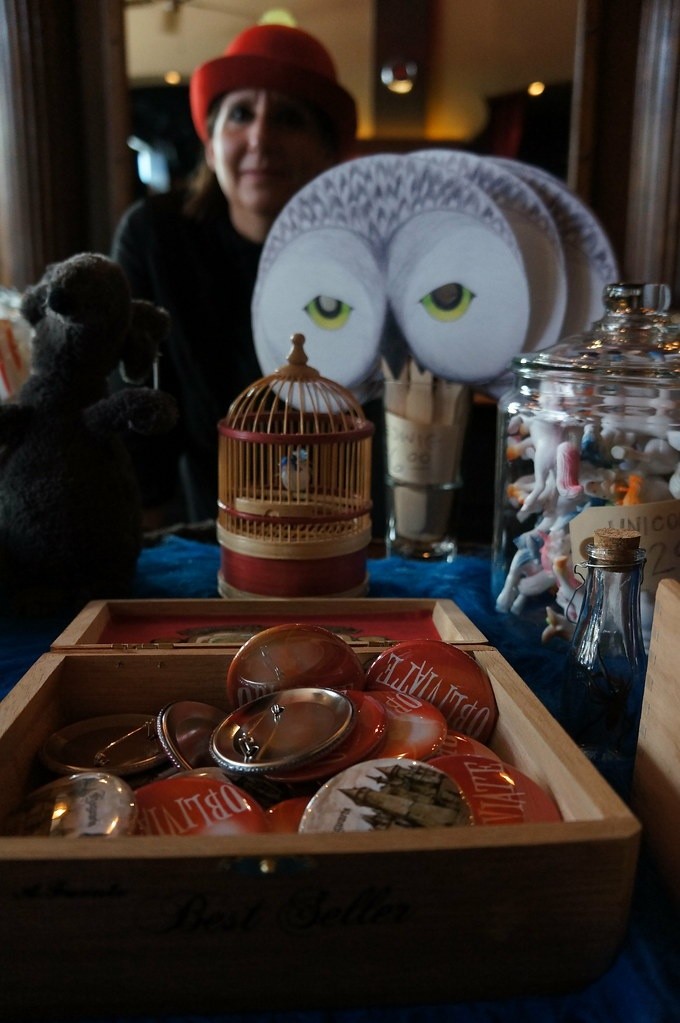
[191,24,357,164]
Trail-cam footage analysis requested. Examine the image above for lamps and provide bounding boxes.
[375,42,417,94]
[160,0,185,38]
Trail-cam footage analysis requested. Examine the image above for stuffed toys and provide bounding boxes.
[0,250,177,575]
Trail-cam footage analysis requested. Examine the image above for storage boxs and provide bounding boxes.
[0,594,640,1023]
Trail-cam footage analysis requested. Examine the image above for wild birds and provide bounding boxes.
[281,449,309,492]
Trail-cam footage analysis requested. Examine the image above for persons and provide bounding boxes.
[114,25,356,530]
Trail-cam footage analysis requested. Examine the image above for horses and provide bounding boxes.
[495,378,680,656]
